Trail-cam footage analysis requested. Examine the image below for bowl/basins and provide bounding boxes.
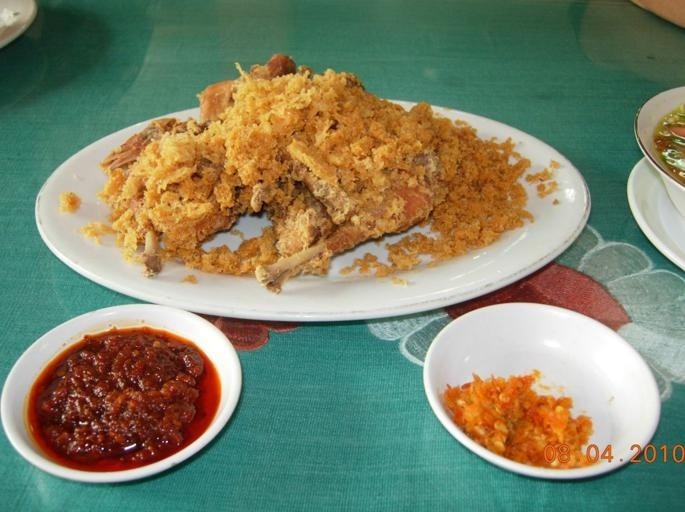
[635,87,685,214]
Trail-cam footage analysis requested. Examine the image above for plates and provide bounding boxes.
[0,304,241,489]
[627,155,685,280]
[429,301,661,484]
[34,97,585,327]
